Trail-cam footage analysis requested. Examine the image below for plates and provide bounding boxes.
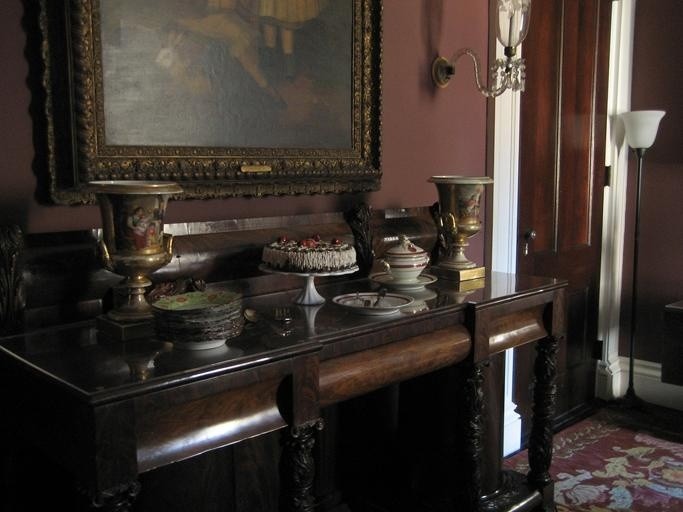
[332,272,439,318]
[148,289,244,344]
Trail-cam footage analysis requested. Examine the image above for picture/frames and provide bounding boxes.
[32,1,385,208]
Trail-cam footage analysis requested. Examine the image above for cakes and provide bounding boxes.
[262,234,356,272]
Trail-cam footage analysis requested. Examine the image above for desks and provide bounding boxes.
[0,266,567,511]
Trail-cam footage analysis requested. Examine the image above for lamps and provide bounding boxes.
[432,0,533,101]
[614,106,666,409]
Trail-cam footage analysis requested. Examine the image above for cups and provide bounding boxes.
[376,232,432,282]
